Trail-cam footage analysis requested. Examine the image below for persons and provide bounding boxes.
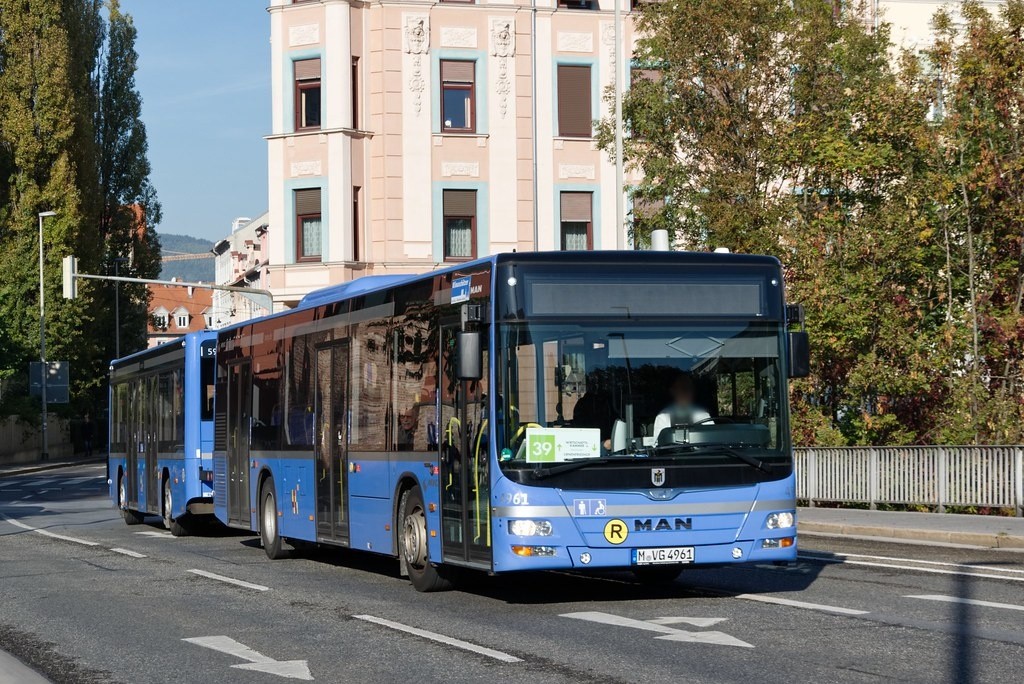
[654,376,713,446]
[396,402,417,451]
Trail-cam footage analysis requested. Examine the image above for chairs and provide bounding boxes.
[271,401,314,446]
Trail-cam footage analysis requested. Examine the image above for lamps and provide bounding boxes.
[209,240,230,257]
[254,224,269,238]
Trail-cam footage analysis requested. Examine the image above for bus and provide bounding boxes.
[108,329,220,536]
[216,252,810,595]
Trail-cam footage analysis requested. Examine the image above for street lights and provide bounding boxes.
[37,210,58,459]
